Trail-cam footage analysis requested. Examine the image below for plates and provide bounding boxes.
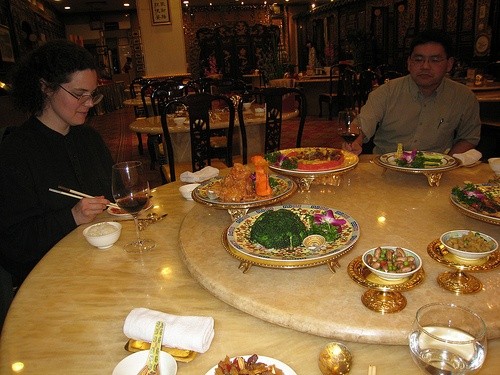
[226,204,361,262]
[112,350,177,375]
[451,183,500,221]
[194,174,294,205]
[379,151,457,171]
[269,147,359,174]
[206,355,297,375]
[107,202,153,216]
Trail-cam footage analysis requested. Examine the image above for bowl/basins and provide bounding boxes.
[243,103,251,110]
[174,117,186,124]
[362,246,422,279]
[439,229,498,260]
[179,183,201,200]
[488,158,500,176]
[82,221,122,249]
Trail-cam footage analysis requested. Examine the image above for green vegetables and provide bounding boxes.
[395,151,425,169]
[306,214,340,243]
[451,183,493,208]
[268,176,279,188]
[265,149,299,170]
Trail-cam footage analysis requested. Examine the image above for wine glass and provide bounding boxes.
[337,111,360,152]
[110,161,157,253]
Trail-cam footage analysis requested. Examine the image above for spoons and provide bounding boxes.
[318,341,352,375]
[138,320,166,375]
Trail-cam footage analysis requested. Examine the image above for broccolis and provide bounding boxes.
[250,209,308,250]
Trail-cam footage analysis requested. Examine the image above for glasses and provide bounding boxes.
[59,84,104,107]
[409,51,448,63]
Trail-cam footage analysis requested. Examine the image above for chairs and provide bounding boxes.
[128,64,403,185]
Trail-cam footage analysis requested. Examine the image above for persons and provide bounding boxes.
[342,29,481,155]
[0,40,128,283]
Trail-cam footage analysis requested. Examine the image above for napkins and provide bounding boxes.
[122,307,215,354]
[180,166,219,183]
[453,149,483,165]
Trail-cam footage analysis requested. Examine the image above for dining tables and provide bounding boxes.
[131,101,299,175]
[140,73,190,82]
[294,78,340,116]
[0,155,500,375]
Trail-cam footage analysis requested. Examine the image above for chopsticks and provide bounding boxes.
[368,366,377,375]
[48,185,121,209]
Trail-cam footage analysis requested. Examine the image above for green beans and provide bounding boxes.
[377,249,408,272]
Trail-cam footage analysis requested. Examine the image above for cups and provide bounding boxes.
[408,302,487,375]
